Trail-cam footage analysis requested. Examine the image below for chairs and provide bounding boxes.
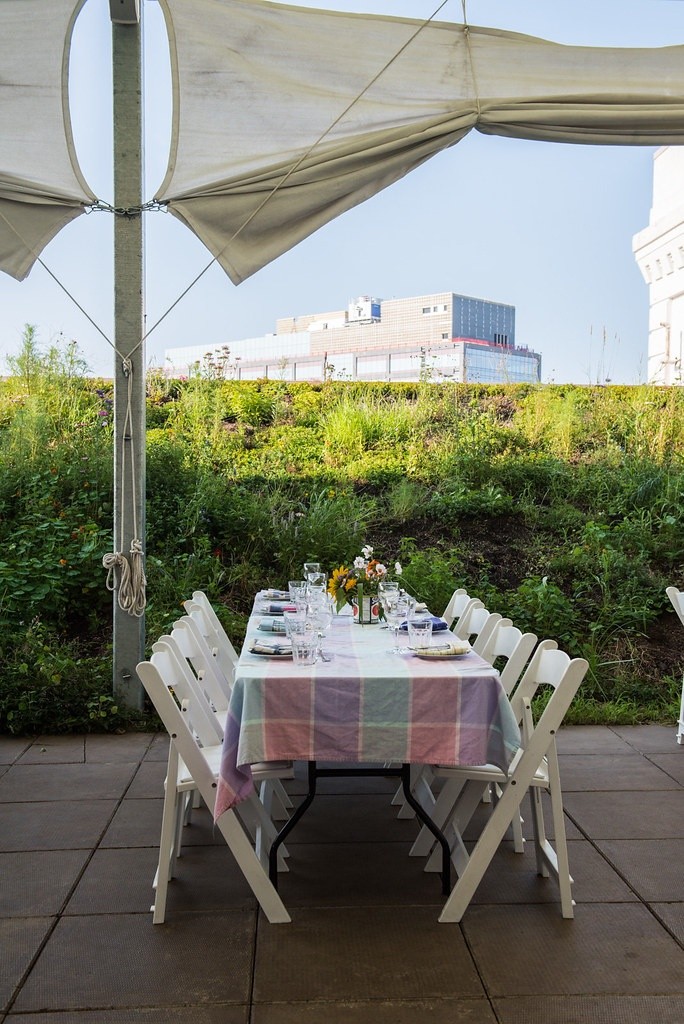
[666,587,684,745]
[382,588,589,922]
[135,590,297,923]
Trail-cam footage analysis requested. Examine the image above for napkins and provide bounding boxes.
[257,617,287,632]
[415,603,426,613]
[417,641,467,655]
[266,604,296,612]
[400,617,447,630]
[248,639,292,655]
[261,588,290,600]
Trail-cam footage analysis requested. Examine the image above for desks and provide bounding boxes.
[214,588,522,897]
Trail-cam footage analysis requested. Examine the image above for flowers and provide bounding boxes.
[326,545,403,623]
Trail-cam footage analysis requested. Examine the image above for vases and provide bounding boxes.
[353,596,379,624]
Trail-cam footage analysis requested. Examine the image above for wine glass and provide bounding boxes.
[376,581,417,655]
[304,562,333,663]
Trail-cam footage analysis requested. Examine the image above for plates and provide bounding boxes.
[261,597,290,601]
[247,650,301,659]
[418,652,471,660]
[262,610,297,616]
[403,629,449,634]
[263,630,302,635]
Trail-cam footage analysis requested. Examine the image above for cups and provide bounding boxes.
[290,630,318,668]
[288,581,307,606]
[407,618,433,654]
[283,609,306,640]
